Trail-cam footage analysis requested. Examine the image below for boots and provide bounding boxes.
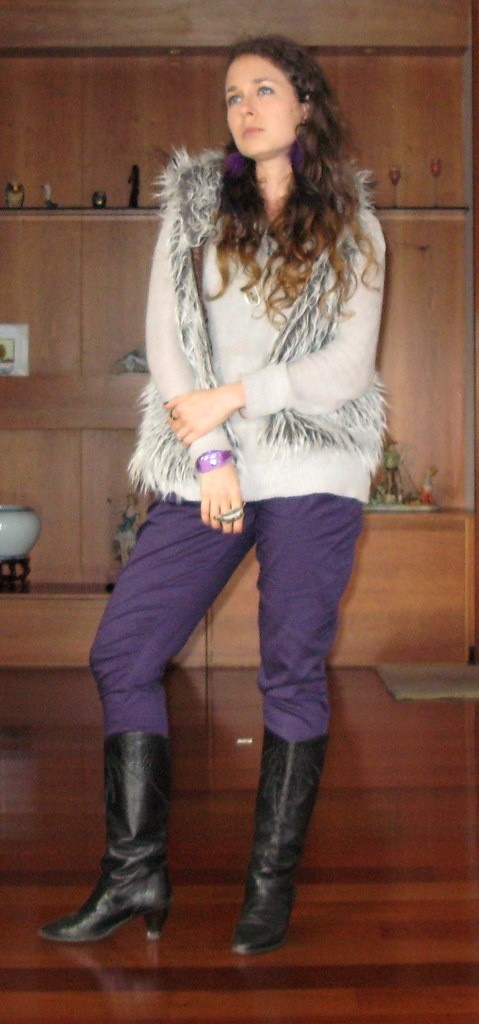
[39,731,174,943]
[228,726,330,955]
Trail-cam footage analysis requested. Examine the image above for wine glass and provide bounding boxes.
[390,165,403,208]
[429,160,443,207]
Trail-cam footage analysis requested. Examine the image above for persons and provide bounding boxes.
[38,34,392,953]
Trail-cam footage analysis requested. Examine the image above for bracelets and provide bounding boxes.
[196,449,232,473]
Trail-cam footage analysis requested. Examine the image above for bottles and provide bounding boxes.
[0,504,41,561]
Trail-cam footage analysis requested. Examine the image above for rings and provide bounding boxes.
[214,498,247,524]
